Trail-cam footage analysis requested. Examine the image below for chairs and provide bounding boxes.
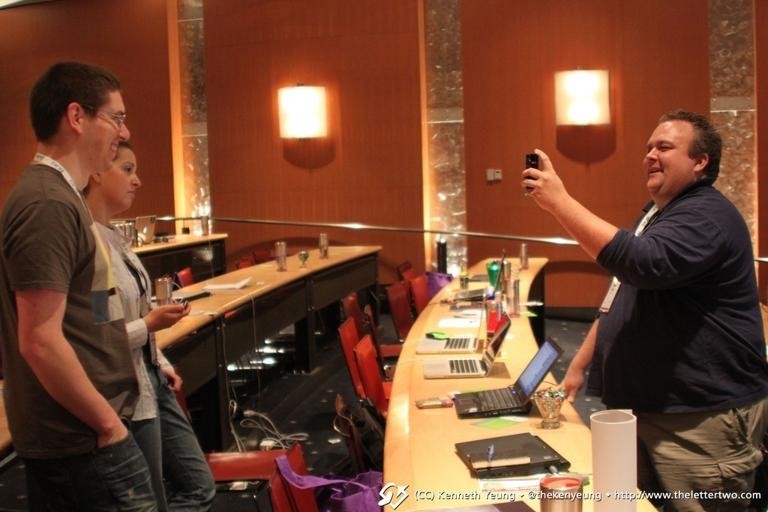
[176,248,435,512]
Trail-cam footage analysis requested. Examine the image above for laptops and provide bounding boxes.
[424,312,511,378]
[151,288,210,302]
[456,253,505,300]
[455,433,570,479]
[470,249,505,281]
[416,300,486,354]
[454,337,563,417]
[135,215,156,244]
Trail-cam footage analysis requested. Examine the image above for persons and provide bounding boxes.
[0,60,217,511]
[522,109,768,512]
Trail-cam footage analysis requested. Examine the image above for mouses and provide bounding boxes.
[426,332,448,339]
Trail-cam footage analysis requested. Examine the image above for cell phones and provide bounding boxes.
[526,154,538,189]
[415,396,453,409]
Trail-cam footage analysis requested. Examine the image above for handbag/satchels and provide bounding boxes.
[274,396,385,510]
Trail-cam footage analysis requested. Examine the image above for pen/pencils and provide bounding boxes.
[488,443,494,463]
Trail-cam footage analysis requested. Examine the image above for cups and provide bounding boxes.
[535,395,566,427]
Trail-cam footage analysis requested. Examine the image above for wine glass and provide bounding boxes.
[297,250,310,267]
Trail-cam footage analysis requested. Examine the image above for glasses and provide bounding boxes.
[82,104,127,126]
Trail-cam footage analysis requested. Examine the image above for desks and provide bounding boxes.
[382,256,661,512]
[133,231,228,297]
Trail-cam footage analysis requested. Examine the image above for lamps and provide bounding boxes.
[278,84,331,140]
[554,69,612,127]
